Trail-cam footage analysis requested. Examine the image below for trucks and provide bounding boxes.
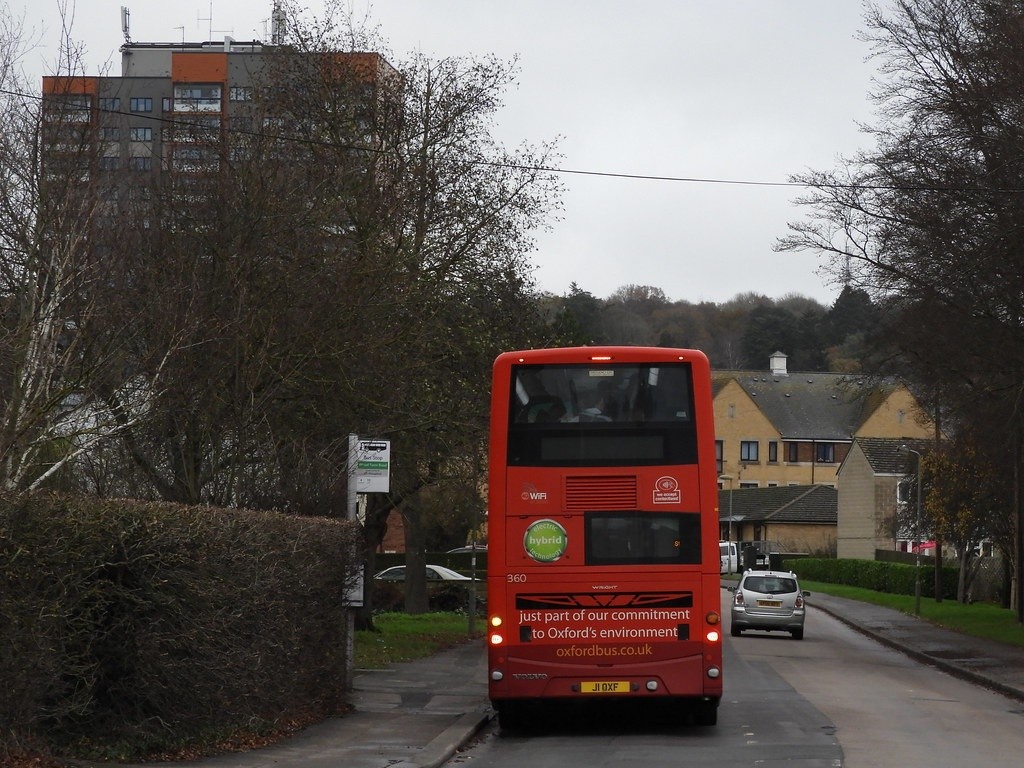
[719,542,770,575]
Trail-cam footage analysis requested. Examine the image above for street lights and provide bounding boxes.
[896,445,923,616]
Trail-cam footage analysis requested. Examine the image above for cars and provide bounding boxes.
[372,563,481,582]
[730,567,811,640]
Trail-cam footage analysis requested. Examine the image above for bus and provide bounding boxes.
[482,344,725,732]
